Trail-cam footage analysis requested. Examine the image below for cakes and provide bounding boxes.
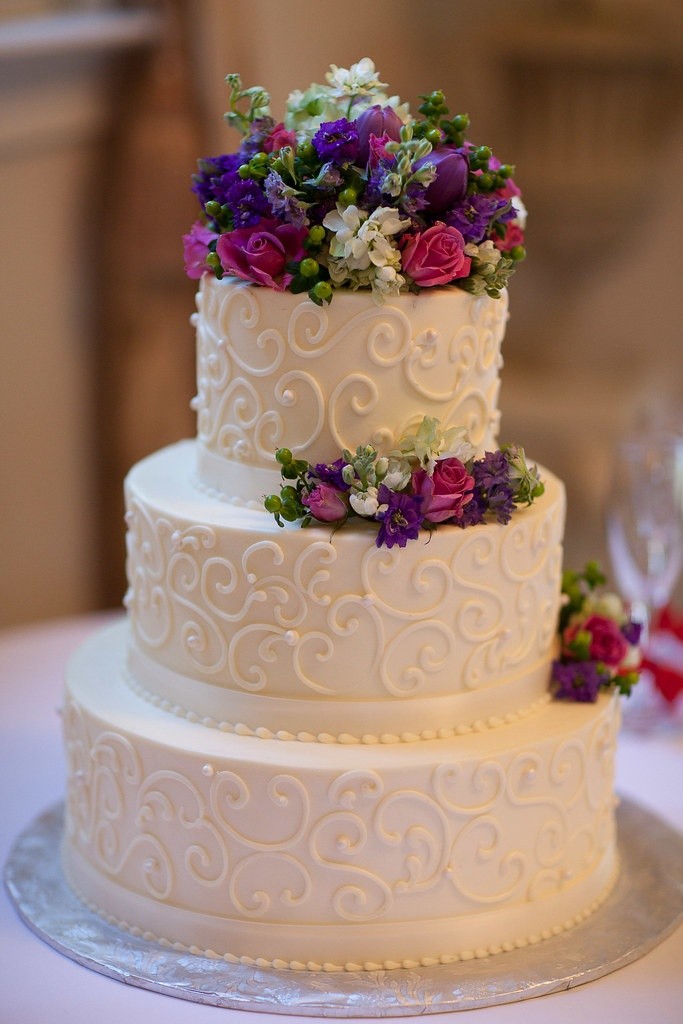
[57,56,644,972]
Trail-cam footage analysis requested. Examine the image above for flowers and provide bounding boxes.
[262,413,546,548]
[180,56,527,308]
[546,559,642,704]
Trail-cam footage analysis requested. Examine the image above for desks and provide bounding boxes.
[0,607,683,1024]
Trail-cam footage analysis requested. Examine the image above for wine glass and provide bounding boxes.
[600,433,682,734]
